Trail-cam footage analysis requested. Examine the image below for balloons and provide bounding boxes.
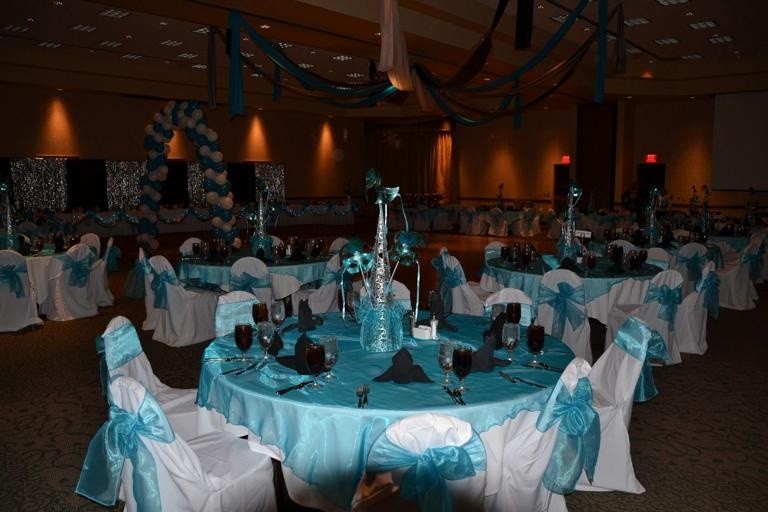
[134,97,245,265]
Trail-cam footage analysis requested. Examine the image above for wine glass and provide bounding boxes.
[305,333,339,395]
[452,346,472,394]
[526,325,543,367]
[346,291,361,323]
[603,225,707,242]
[500,242,537,270]
[501,321,520,365]
[725,222,750,236]
[192,228,324,263]
[235,298,284,364]
[584,244,648,277]
[439,341,459,386]
[32,233,81,257]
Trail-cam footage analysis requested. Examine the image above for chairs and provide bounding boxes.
[497,352,593,512]
[101,315,197,441]
[281,197,467,232]
[573,316,671,495]
[362,414,488,511]
[361,278,414,315]
[105,373,279,511]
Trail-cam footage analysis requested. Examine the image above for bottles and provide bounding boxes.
[429,313,439,341]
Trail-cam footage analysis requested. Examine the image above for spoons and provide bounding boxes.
[510,377,546,389]
[453,389,465,404]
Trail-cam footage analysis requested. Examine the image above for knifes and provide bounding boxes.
[278,380,314,395]
[207,356,257,377]
[442,385,460,405]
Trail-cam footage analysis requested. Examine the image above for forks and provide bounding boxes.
[357,383,371,409]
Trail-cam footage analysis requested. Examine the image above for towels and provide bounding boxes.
[374,346,433,386]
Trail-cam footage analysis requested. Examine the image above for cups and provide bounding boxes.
[491,303,521,323]
